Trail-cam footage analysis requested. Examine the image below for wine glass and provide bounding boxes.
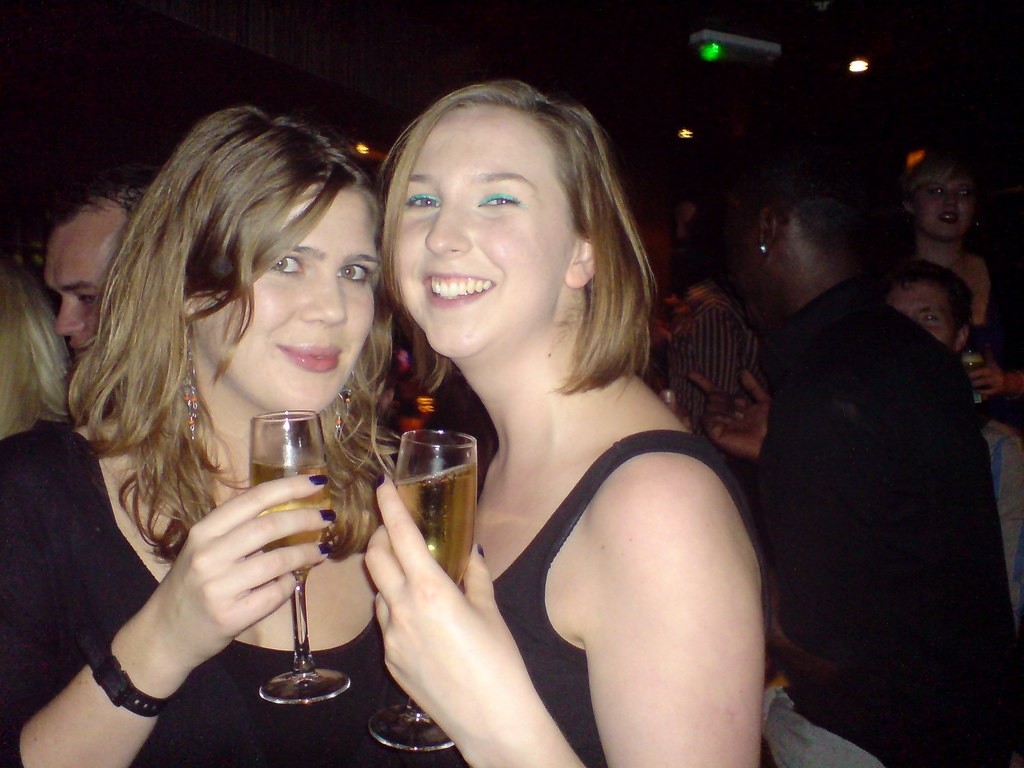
[366,429,478,752]
[249,410,350,704]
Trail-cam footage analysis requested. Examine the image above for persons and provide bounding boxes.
[0,184,155,439]
[0,105,382,768]
[363,81,765,768]
[632,84,1024,768]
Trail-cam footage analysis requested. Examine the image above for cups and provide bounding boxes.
[958,344,994,408]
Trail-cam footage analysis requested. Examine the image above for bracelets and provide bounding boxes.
[77,637,170,717]
